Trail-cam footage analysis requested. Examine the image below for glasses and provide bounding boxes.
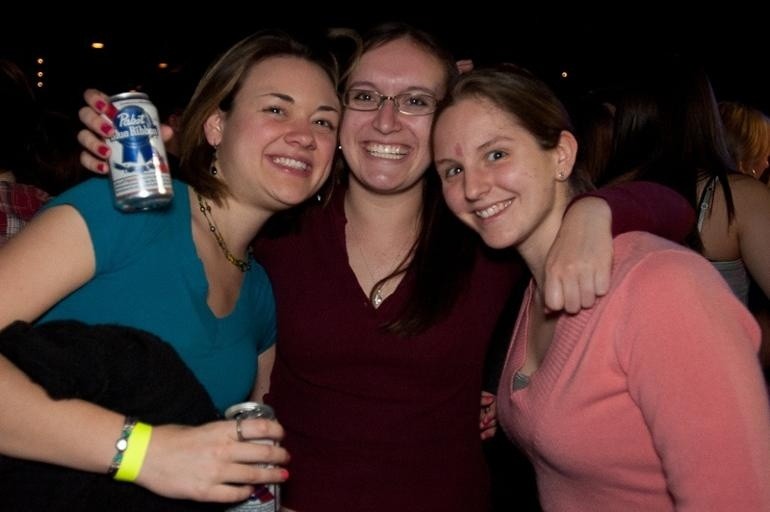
[342,89,438,115]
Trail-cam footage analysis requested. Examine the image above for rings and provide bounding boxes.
[233,414,247,441]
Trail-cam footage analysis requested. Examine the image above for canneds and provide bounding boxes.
[224,401,282,512]
[102,91,175,214]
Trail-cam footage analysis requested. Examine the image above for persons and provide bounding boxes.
[430,64,770,510]
[0,26,366,512]
[560,54,769,314]
[1,67,62,245]
[78,27,695,508]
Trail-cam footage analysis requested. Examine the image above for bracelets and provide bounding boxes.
[114,420,153,484]
[107,415,136,478]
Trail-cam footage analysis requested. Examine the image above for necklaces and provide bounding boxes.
[348,214,413,306]
[195,191,251,274]
